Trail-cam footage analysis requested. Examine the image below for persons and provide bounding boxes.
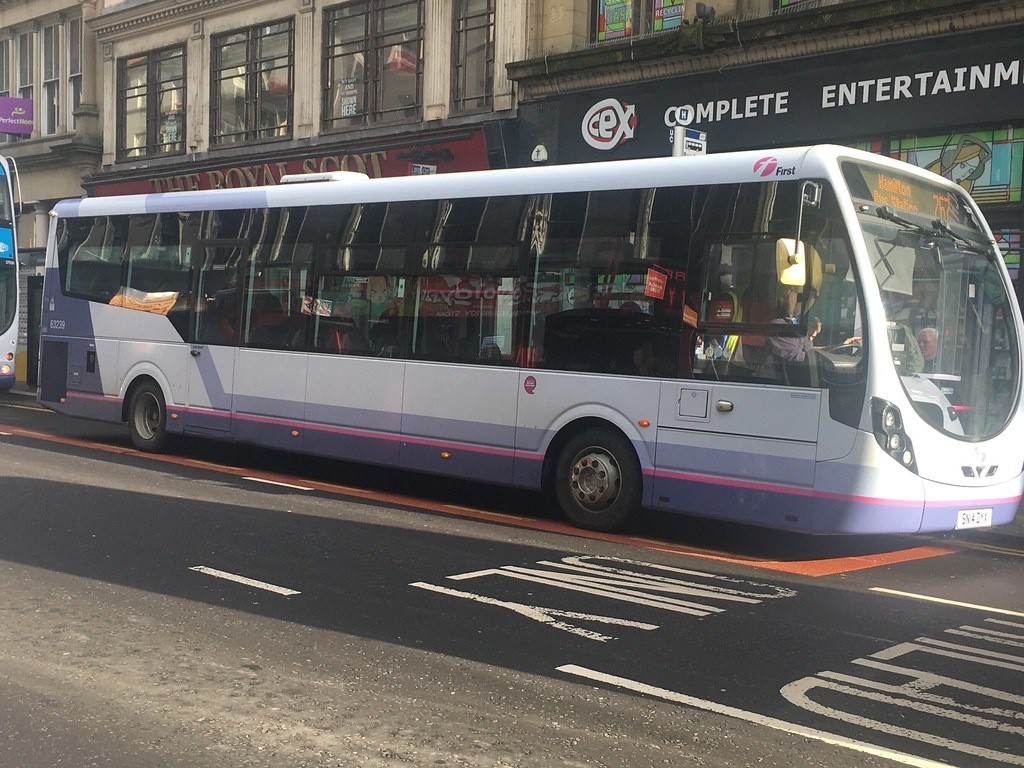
[199,262,956,394]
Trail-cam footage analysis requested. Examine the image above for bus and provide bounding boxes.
[38,142,1024,536]
[0,153,22,389]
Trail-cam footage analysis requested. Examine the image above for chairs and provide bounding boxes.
[212,265,774,380]
